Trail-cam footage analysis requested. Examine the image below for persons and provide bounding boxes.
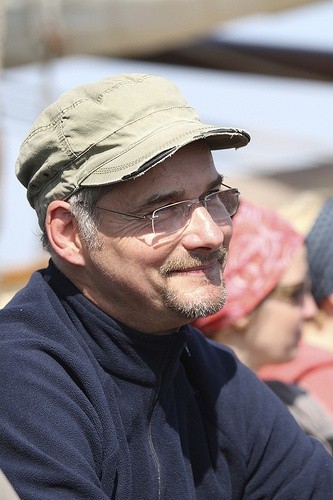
[251,191,332,421]
[0,74,331,500]
[183,186,332,461]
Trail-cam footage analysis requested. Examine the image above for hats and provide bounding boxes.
[15,73,250,227]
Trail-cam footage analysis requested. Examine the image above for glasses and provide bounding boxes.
[90,183,242,235]
